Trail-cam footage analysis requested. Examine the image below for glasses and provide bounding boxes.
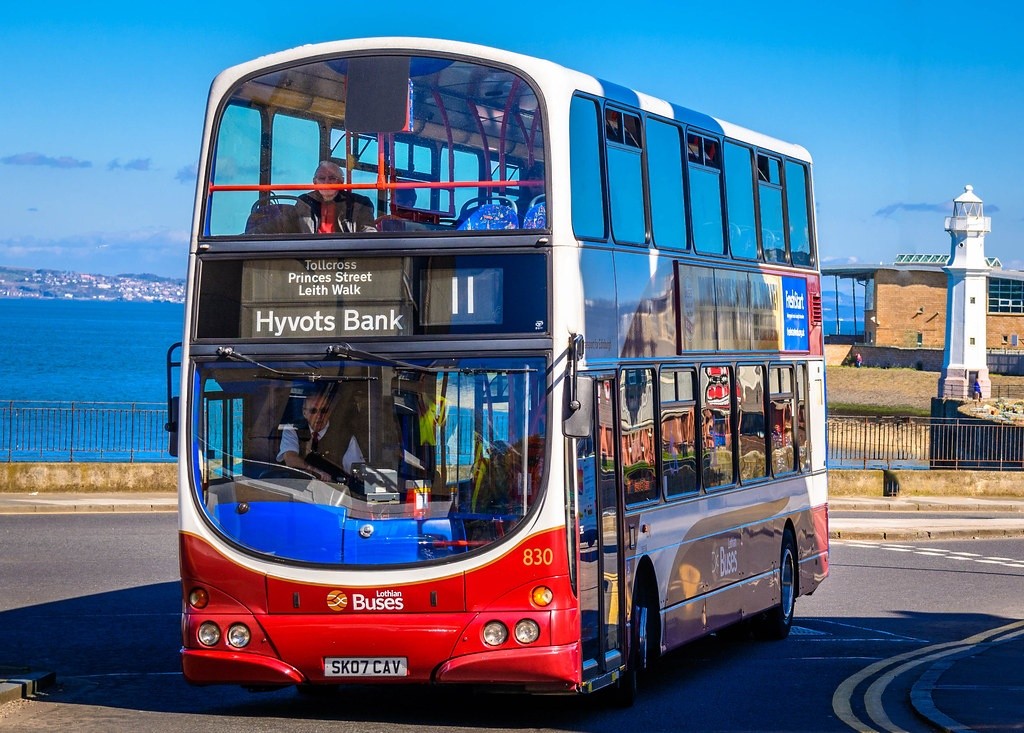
[315,175,343,182]
[302,407,330,415]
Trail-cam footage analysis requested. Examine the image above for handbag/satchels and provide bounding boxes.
[246,195,316,234]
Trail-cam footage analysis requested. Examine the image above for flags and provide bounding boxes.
[414,372,490,511]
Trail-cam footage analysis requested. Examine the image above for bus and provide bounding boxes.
[163,35,834,707]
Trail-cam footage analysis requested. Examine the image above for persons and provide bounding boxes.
[294,161,376,235]
[973,377,982,402]
[854,353,862,368]
[272,381,366,482]
[771,423,783,449]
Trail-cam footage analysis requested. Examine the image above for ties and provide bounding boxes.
[310,431,320,452]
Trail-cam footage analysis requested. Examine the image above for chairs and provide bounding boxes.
[602,446,808,510]
[245,194,547,234]
